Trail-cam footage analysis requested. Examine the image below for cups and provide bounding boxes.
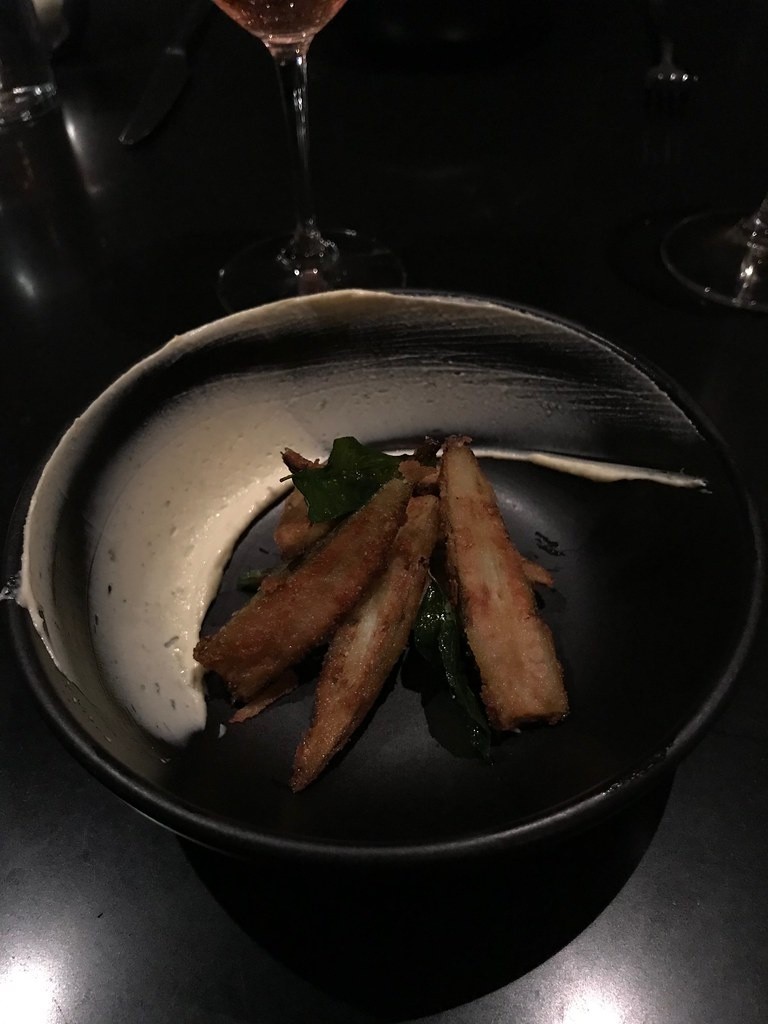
[0,0,63,135]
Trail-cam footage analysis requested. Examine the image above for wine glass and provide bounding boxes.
[658,189,768,312]
[211,0,407,313]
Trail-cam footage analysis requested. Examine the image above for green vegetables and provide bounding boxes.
[237,437,501,768]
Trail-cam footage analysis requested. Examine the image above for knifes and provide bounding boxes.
[117,0,212,146]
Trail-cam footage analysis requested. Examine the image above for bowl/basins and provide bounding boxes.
[2,288,766,862]
